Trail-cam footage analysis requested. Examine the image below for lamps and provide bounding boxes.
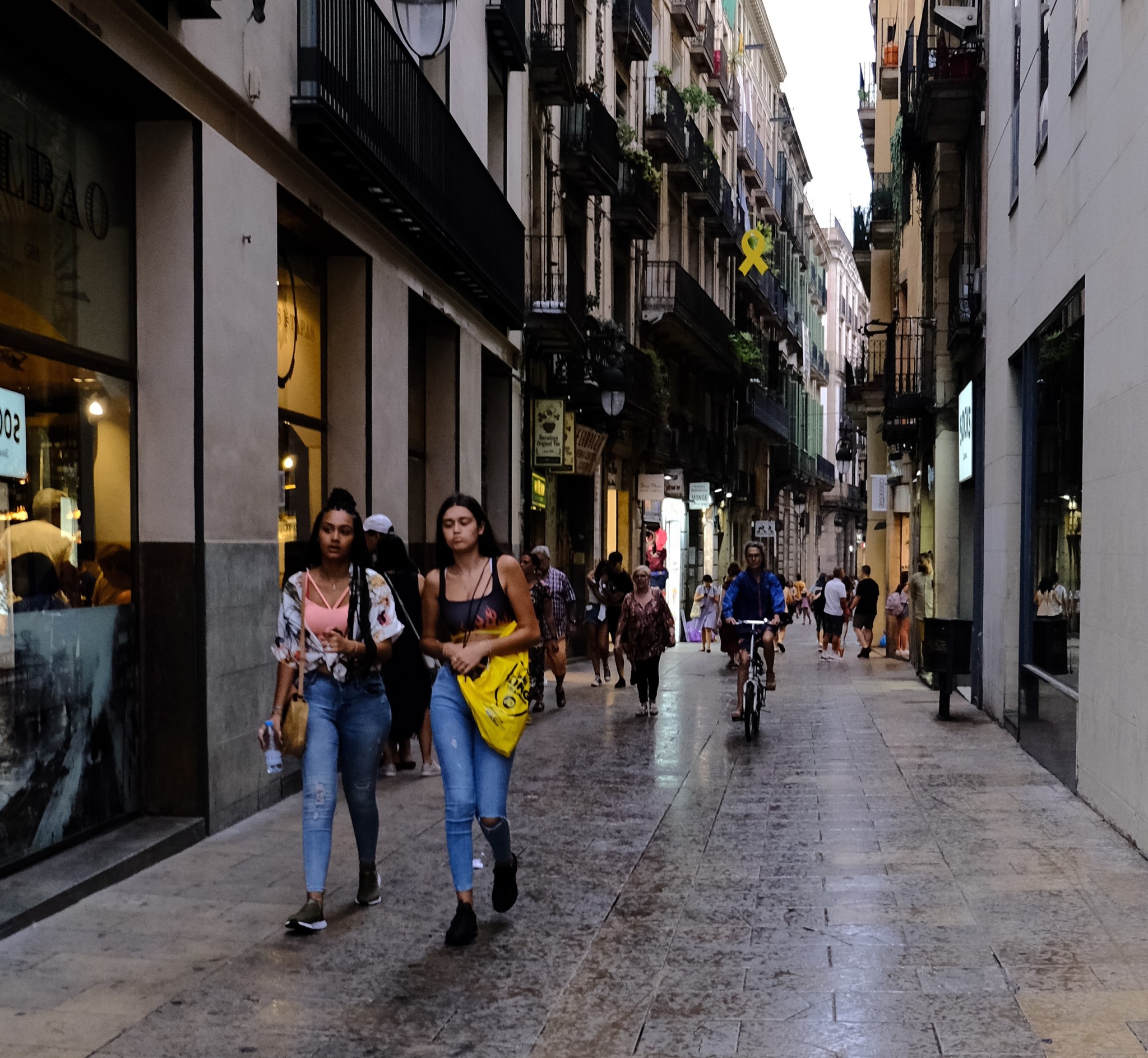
[793,493,805,514]
[601,386,625,417]
[834,510,845,533]
[855,522,864,534]
[713,482,722,493]
[391,0,456,60]
[831,423,868,475]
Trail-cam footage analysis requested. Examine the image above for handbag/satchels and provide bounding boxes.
[663,617,676,647]
[455,619,531,756]
[779,612,794,624]
[689,601,701,618]
[276,683,309,756]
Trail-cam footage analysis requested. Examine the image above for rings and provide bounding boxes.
[451,656,458,662]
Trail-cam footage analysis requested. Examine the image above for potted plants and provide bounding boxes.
[650,64,721,172]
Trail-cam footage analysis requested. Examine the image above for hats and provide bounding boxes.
[362,514,395,534]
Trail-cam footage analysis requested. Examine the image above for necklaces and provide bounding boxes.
[328,581,336,591]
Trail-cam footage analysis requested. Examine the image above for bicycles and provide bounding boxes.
[732,617,779,743]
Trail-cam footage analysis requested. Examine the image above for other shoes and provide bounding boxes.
[591,674,602,686]
[635,699,658,717]
[775,642,785,653]
[630,666,637,685]
[395,760,416,772]
[603,663,611,682]
[922,672,933,680]
[817,643,873,662]
[491,851,518,913]
[421,759,442,776]
[383,762,397,776]
[442,901,478,945]
[731,706,742,717]
[615,678,626,688]
[766,671,776,688]
[532,700,545,712]
[355,869,381,905]
[285,898,328,929]
[725,660,740,669]
[555,685,566,708]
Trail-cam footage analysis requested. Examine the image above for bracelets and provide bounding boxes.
[272,706,283,710]
[272,711,282,716]
[486,639,492,657]
[353,641,358,655]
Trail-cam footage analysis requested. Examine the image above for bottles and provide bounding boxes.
[262,720,284,774]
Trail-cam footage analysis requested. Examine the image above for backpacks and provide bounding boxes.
[885,592,908,616]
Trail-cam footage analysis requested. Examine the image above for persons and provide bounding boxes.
[1032,571,1070,673]
[254,487,938,951]
[0,487,136,616]
